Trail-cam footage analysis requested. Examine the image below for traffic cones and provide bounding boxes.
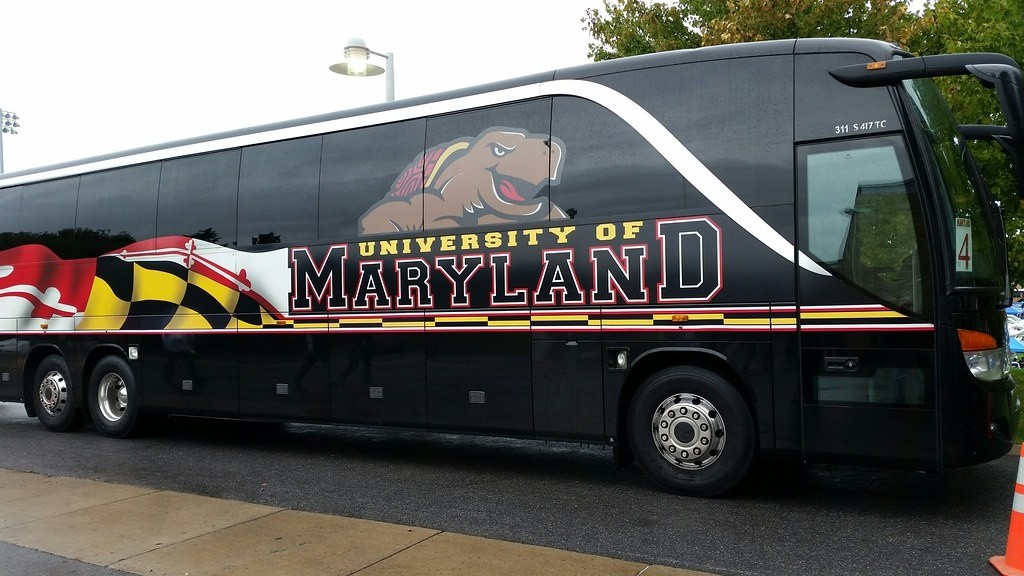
[988,440,1024,576]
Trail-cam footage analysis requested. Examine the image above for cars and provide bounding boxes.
[1011,361,1021,370]
[1002,301,1024,354]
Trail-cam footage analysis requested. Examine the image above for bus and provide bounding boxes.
[0,37,1024,499]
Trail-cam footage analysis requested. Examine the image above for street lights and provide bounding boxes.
[328,38,394,104]
[0,108,20,174]
